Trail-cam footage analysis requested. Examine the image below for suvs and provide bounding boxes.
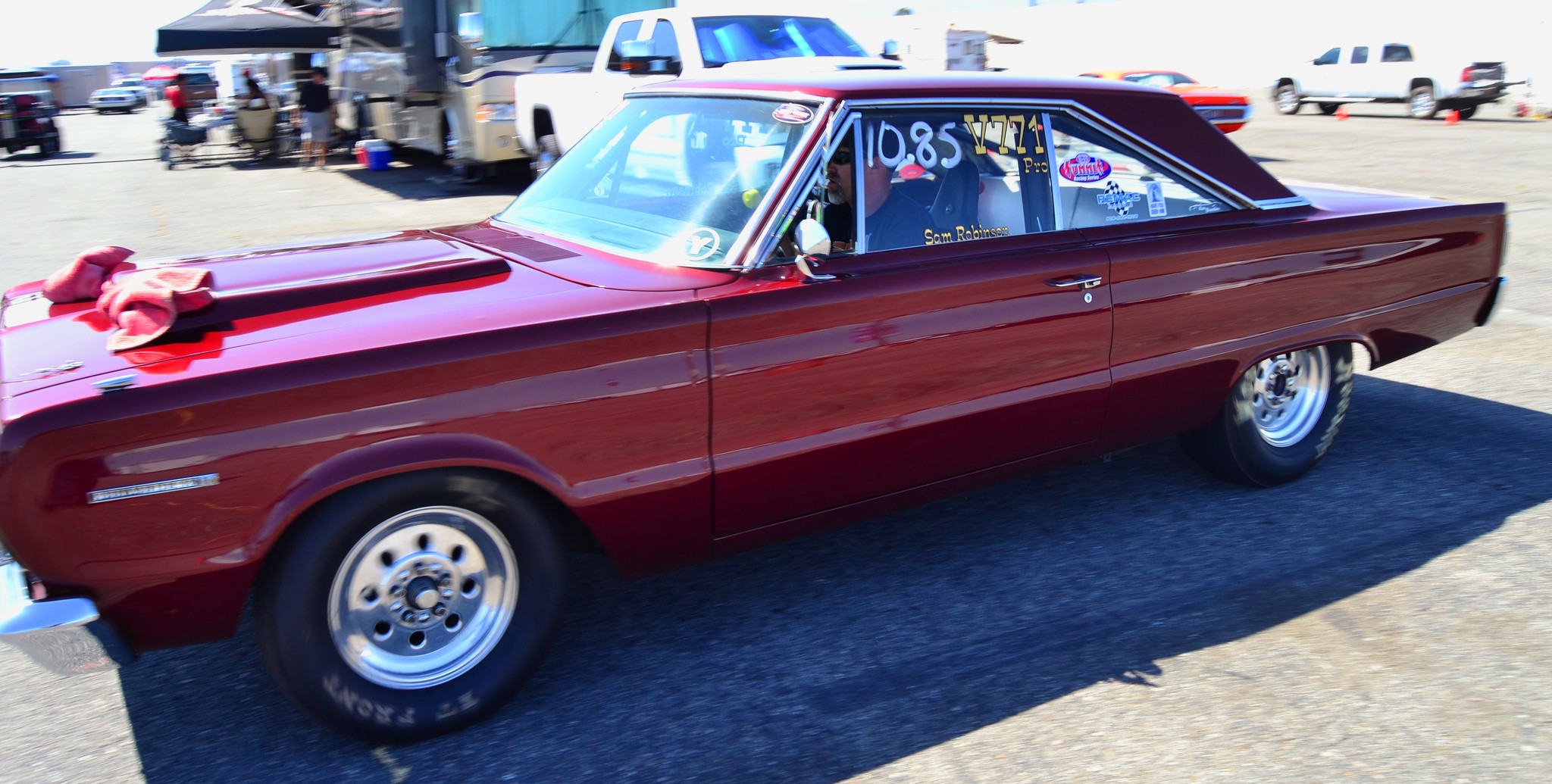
[1,92,63,156]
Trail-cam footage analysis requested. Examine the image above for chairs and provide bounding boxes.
[923,155,1219,239]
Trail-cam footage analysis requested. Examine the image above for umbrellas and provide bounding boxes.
[141,65,179,99]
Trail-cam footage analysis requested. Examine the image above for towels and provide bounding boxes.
[41,244,136,302]
[98,264,213,355]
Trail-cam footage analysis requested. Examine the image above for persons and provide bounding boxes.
[775,116,938,259]
[170,75,195,126]
[242,69,266,97]
[296,67,335,166]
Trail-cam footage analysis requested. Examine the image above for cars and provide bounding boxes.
[1075,69,1251,135]
[87,65,220,112]
[0,57,1510,747]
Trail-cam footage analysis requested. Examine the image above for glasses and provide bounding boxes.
[832,151,850,165]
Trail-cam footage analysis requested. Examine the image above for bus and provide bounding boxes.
[329,1,679,179]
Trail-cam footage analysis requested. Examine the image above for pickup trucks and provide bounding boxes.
[510,6,908,201]
[1270,41,1509,120]
